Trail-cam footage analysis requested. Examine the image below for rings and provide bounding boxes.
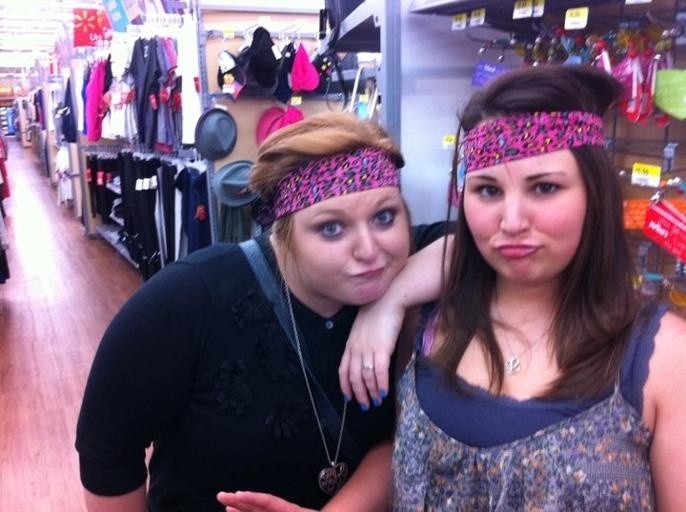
[363,359,377,376]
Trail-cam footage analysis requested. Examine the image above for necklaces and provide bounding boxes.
[490,281,561,378]
[283,254,350,495]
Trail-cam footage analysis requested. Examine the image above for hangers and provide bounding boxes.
[145,10,195,30]
[83,142,207,174]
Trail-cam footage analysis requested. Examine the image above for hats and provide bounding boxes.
[216,24,323,103]
[256,105,306,148]
[213,160,263,209]
[196,108,237,161]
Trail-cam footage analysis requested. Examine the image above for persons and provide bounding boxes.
[72,110,463,512]
[216,58,686,512]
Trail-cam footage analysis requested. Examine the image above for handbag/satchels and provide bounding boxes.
[319,0,365,47]
